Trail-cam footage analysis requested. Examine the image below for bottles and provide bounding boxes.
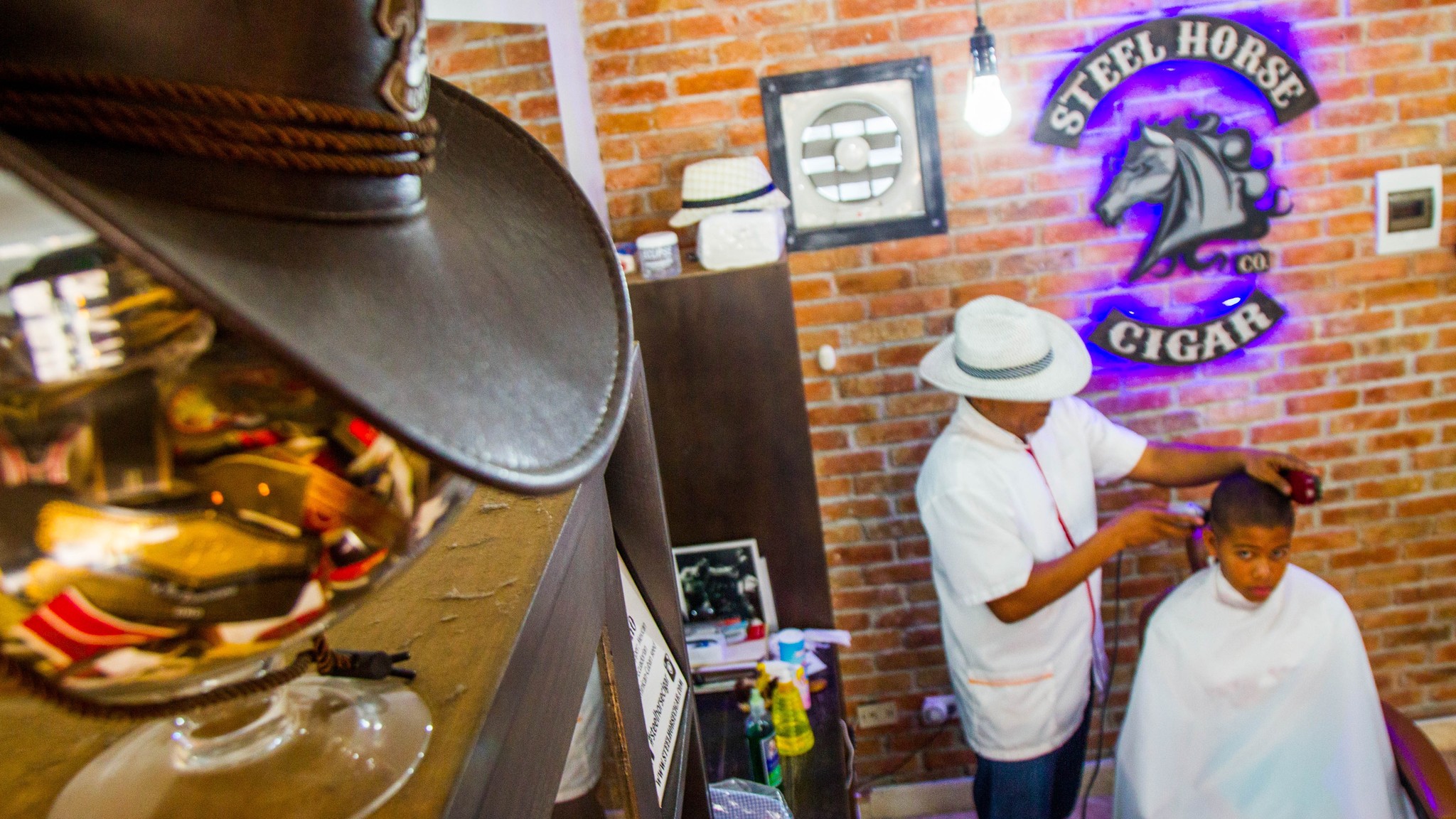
[615,230,682,279]
[744,628,815,788]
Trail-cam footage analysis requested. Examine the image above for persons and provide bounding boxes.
[914,293,1323,818]
[680,548,757,616]
[1113,474,1409,819]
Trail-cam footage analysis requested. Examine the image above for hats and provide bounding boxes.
[916,295,1092,403]
[669,156,795,232]
[0,0,637,496]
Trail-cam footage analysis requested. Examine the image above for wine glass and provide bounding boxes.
[0,169,476,819]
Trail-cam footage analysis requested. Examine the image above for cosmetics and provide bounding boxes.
[745,689,784,789]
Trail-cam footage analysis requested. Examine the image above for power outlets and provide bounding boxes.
[923,697,960,719]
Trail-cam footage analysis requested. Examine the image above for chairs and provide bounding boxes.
[1140,517,1456,819]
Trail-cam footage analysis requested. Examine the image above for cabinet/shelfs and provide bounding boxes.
[0,343,717,819]
